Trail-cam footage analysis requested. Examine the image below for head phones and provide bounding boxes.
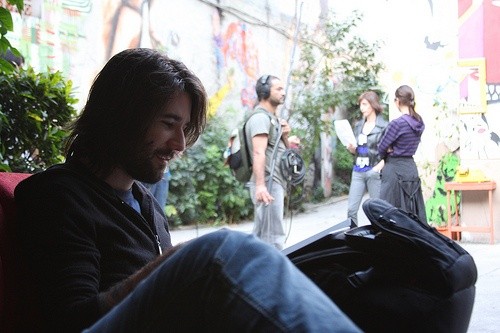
[259,75,271,99]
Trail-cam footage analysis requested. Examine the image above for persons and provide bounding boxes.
[346,91,390,231]
[377,85,428,231]
[14,49,363,333]
[244,73,299,254]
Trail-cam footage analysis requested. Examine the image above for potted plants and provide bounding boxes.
[425,153,460,239]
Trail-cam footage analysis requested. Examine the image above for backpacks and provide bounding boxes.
[286,198,477,333]
[223,109,274,182]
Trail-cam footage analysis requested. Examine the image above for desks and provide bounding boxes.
[445,181,496,244]
